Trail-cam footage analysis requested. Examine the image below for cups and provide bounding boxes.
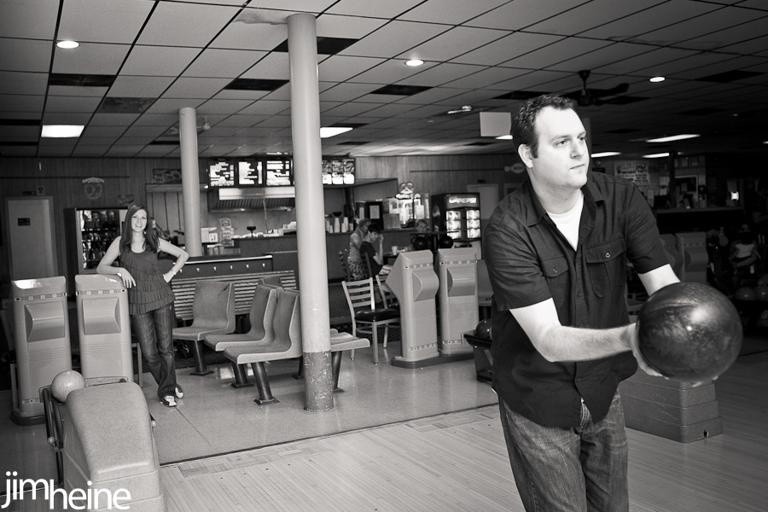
[325,218,349,233]
[392,245,398,256]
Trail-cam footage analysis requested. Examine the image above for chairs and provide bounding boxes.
[173,246,399,405]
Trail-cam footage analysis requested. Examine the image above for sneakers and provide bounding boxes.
[175,385,184,398]
[161,395,177,407]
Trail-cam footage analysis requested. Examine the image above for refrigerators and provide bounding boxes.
[432,192,481,242]
[65,206,129,271]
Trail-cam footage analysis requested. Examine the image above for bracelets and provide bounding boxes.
[169,269,177,276]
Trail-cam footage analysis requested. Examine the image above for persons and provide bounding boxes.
[360,223,395,296]
[346,216,371,309]
[94,201,191,407]
[729,230,763,277]
[729,220,768,294]
[400,219,427,251]
[482,89,719,512]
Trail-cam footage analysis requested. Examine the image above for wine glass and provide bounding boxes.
[247,219,258,238]
